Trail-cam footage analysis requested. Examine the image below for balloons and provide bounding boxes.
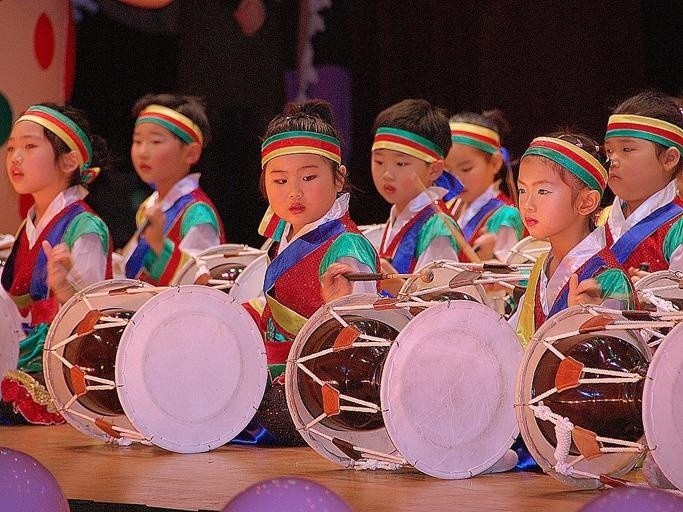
[576,486,683,512]
[0,447,70,511]
[221,477,353,511]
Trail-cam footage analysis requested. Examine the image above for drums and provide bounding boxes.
[505,232,553,269]
[634,267,683,305]
[43,278,269,454]
[514,303,683,500]
[173,243,270,303]
[353,221,387,252]
[284,292,524,480]
[397,259,531,329]
[1,286,21,393]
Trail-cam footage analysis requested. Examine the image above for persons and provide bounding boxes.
[114,89,226,292]
[503,132,641,474]
[601,86,682,296]
[445,108,525,262]
[1,101,113,424]
[369,98,479,298]
[232,98,378,447]
